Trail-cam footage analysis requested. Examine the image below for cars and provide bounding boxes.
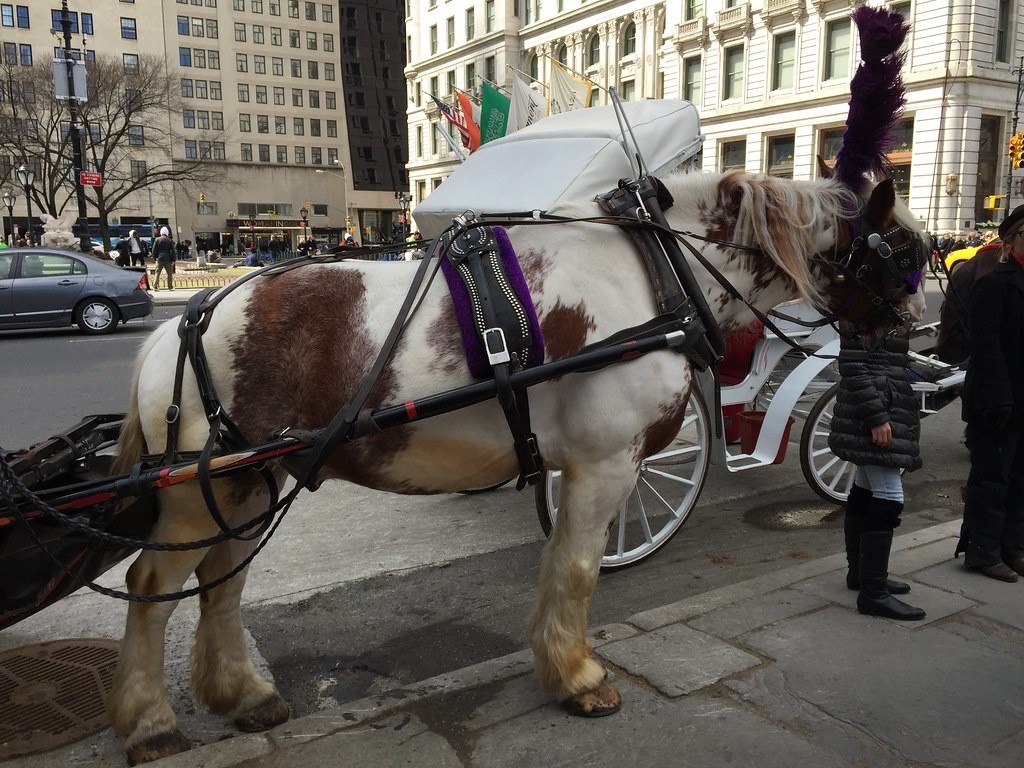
[943,235,1004,279]
[0,248,154,335]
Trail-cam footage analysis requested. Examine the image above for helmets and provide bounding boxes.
[159,227,170,237]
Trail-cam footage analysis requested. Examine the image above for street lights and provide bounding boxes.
[300,206,309,244]
[398,194,409,243]
[315,160,349,236]
[155,219,159,236]
[249,213,256,249]
[16,161,36,245]
[1,191,16,245]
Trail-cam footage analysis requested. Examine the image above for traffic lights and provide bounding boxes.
[987,197,999,210]
[346,216,351,227]
[1010,138,1017,159]
[200,193,205,204]
[1013,132,1024,171]
[349,227,356,233]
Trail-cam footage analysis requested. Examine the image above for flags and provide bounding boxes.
[430,73,547,157]
[547,59,592,117]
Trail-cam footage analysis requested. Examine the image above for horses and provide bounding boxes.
[97,153,933,768]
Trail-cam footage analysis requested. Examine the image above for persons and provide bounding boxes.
[371,218,420,260]
[827,226,926,621]
[929,205,1024,582]
[113,226,190,292]
[238,237,359,267]
[0,233,30,249]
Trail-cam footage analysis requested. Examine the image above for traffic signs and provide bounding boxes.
[80,171,101,187]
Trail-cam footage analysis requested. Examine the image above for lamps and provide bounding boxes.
[946,170,957,196]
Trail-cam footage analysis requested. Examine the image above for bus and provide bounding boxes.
[31,224,157,258]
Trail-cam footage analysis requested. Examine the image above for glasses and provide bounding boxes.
[1017,231,1024,240]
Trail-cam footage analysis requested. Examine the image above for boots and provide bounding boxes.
[844,480,911,595]
[857,497,927,620]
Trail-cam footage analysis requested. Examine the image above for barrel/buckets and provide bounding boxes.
[738,411,795,464]
[722,404,745,444]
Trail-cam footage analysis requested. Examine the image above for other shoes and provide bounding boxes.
[964,552,1018,582]
[1002,550,1024,576]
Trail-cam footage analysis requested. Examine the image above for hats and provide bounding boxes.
[998,204,1024,240]
[344,233,352,241]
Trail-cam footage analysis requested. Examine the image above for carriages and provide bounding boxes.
[411,100,1010,575]
[0,155,936,757]
